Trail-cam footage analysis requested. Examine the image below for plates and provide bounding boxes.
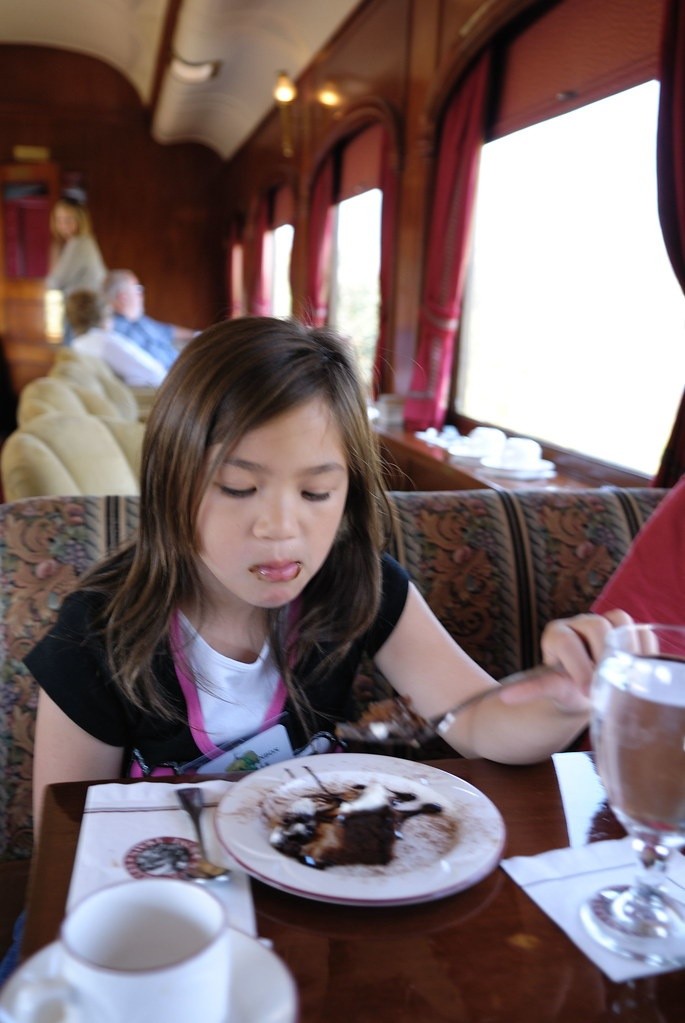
[481,456,556,473]
[447,443,500,459]
[213,754,505,905]
[1,926,297,1023]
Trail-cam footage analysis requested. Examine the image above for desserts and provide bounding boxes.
[308,780,394,866]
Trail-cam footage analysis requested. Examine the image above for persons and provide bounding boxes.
[45,198,106,347]
[104,269,197,386]
[592,476,684,656]
[1,318,659,983]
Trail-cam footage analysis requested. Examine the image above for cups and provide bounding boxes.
[12,876,229,1023]
[505,436,542,467]
[468,426,506,453]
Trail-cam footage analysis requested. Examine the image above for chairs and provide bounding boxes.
[0,347,150,502]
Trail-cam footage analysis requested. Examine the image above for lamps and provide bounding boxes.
[272,70,375,109]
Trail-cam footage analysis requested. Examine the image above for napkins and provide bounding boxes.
[496,749,685,981]
[66,779,260,937]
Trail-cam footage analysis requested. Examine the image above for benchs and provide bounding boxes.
[0,484,667,896]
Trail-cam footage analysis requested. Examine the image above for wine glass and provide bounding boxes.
[578,623,685,959]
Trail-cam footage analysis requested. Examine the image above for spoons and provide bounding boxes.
[176,787,231,884]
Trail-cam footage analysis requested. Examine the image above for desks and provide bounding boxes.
[17,755,685,1023]
